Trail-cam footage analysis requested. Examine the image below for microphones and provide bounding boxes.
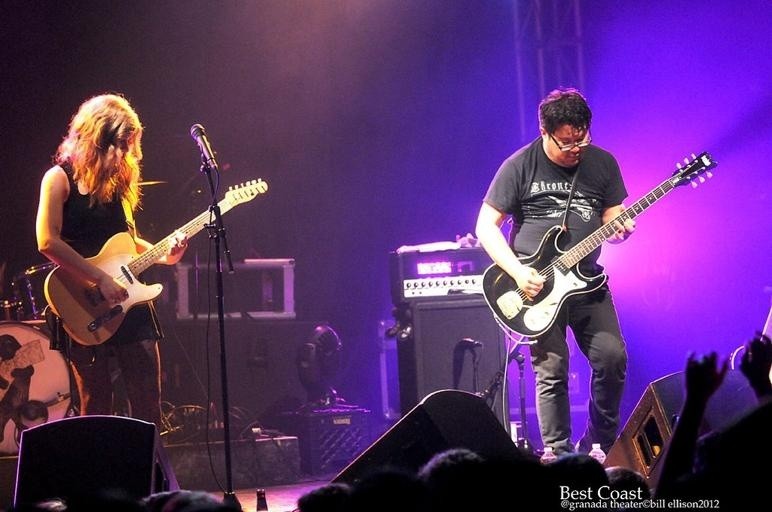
[190,124,217,171]
[460,337,482,348]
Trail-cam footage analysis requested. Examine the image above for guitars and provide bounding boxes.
[482,150,719,339]
[43,177,268,347]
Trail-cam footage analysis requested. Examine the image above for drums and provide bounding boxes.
[0,320,80,460]
[10,262,58,323]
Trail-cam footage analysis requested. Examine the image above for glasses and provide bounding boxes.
[542,124,593,153]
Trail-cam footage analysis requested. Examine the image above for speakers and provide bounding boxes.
[602,370,761,494]
[328,389,521,489]
[397,299,511,440]
[11,415,157,512]
[299,414,372,477]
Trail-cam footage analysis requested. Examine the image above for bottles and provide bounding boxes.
[588,442,607,465]
[256,487,268,511]
[540,446,558,466]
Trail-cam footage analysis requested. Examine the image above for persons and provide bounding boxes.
[21,489,242,512]
[654,333,772,501]
[35,92,189,431]
[474,86,628,453]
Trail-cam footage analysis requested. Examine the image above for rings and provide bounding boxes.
[123,291,128,297]
